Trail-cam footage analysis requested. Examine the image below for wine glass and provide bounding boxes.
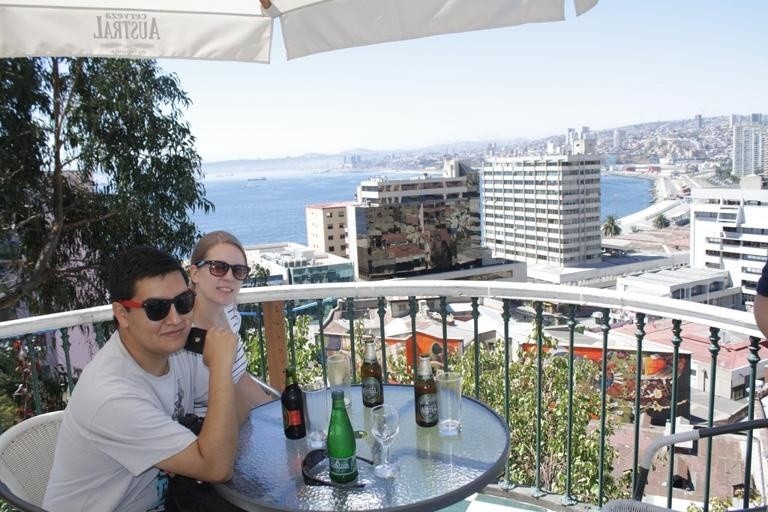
[368,403,401,482]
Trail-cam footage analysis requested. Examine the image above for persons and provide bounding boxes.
[42,248,251,512]
[188,230,272,421]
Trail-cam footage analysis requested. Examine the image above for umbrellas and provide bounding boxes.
[0,0,600,65]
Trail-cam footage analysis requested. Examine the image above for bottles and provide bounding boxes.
[360,339,383,407]
[280,367,306,441]
[414,351,439,428]
[328,391,358,483]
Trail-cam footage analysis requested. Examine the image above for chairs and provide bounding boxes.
[1,408,70,511]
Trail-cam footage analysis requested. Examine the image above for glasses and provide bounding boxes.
[301,450,373,488]
[118,289,197,321]
[197,260,251,280]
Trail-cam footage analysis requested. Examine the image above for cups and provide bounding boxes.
[328,356,353,410]
[434,370,463,436]
